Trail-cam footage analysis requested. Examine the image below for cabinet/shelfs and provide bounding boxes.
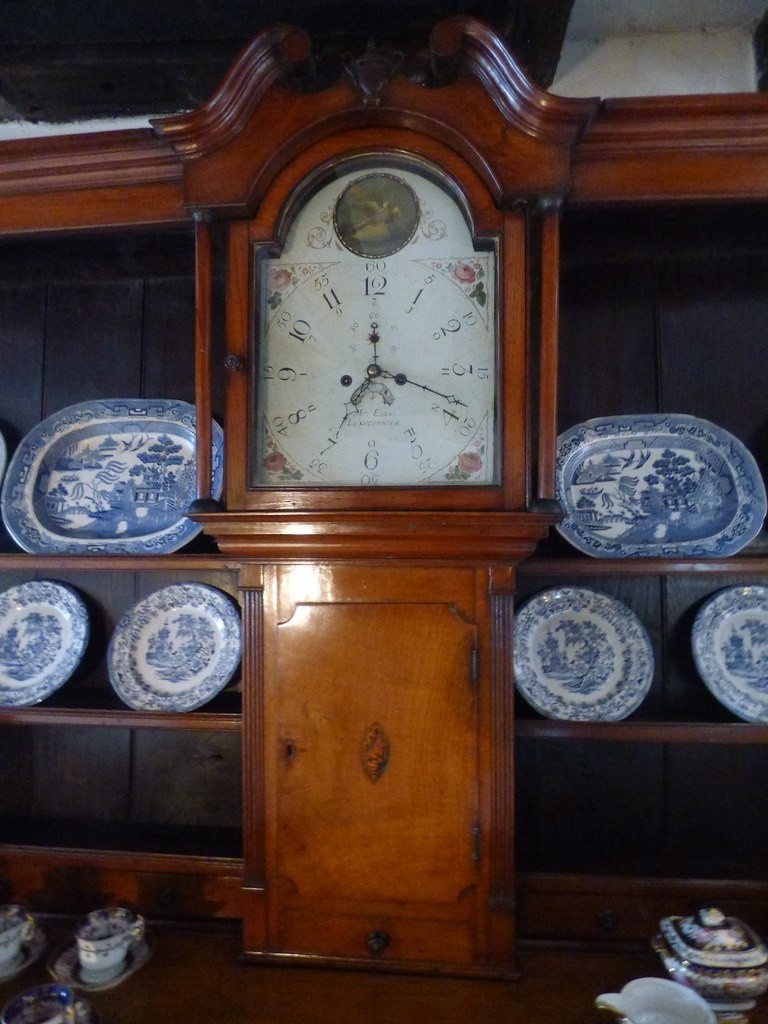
[0,94,768,903]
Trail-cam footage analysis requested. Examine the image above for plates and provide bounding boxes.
[0,580,89,708]
[1,399,224,555]
[692,584,768,723]
[74,998,103,1024]
[106,582,240,714]
[513,586,655,723]
[554,415,767,559]
[0,923,45,982]
[50,928,155,992]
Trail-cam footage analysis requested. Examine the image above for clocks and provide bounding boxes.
[147,16,602,982]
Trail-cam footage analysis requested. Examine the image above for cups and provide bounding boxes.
[72,907,143,971]
[595,977,717,1024]
[0,905,35,963]
[0,983,74,1024]
